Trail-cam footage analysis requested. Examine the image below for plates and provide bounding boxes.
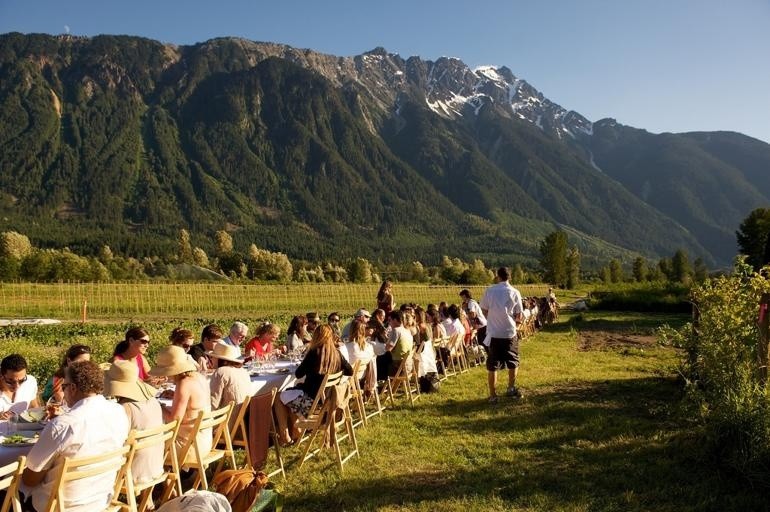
[13,423,42,430]
[0,437,39,446]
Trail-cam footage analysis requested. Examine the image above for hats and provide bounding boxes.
[205,341,244,363]
[98,360,158,402]
[306,312,323,322]
[355,310,371,317]
[148,345,198,376]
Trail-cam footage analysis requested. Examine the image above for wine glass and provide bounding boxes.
[289,350,304,367]
[248,352,277,375]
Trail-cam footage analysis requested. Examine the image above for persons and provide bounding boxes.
[20,357,130,512]
[345,318,373,387]
[273,323,354,446]
[416,289,488,377]
[0,353,39,422]
[376,309,414,382]
[479,265,524,405]
[223,321,257,366]
[376,279,397,313]
[521,287,557,337]
[187,323,224,374]
[287,315,313,353]
[96,359,165,512]
[400,303,417,336]
[244,321,287,363]
[110,326,152,382]
[167,326,194,354]
[371,308,386,328]
[204,342,252,438]
[41,343,91,407]
[343,309,374,338]
[327,312,342,339]
[306,310,321,338]
[147,345,214,507]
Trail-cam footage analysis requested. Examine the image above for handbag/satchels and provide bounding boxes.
[208,464,268,512]
[157,489,232,512]
[420,374,439,392]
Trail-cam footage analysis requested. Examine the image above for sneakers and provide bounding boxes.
[488,395,497,404]
[507,387,517,395]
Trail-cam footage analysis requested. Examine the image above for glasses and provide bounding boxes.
[238,332,247,339]
[389,286,393,288]
[62,384,71,392]
[138,338,150,344]
[331,319,339,322]
[2,374,27,385]
[69,345,91,353]
[176,340,192,349]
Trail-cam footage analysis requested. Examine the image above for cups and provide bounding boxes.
[7,414,18,435]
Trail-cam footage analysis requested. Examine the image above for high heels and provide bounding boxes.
[278,439,295,446]
